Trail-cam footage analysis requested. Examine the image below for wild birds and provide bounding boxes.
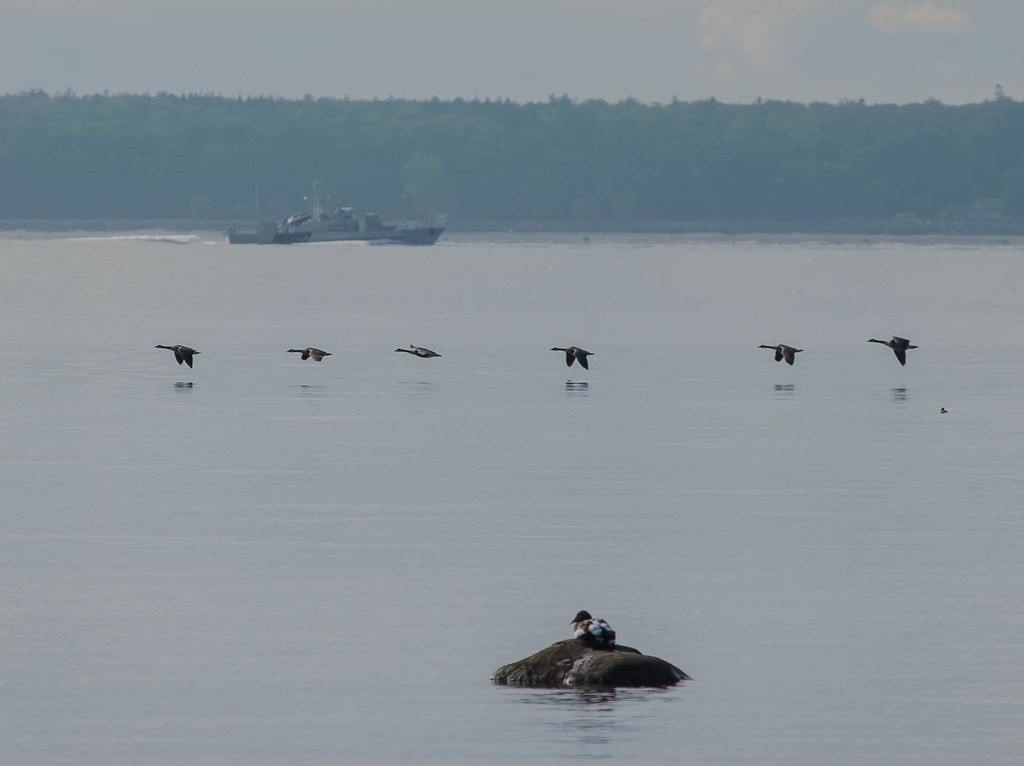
[866,335,920,368]
[756,342,805,367]
[549,345,595,371]
[393,343,443,359]
[153,343,203,370]
[285,345,333,363]
[567,608,617,651]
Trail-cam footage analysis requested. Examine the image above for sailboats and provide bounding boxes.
[225,178,447,247]
